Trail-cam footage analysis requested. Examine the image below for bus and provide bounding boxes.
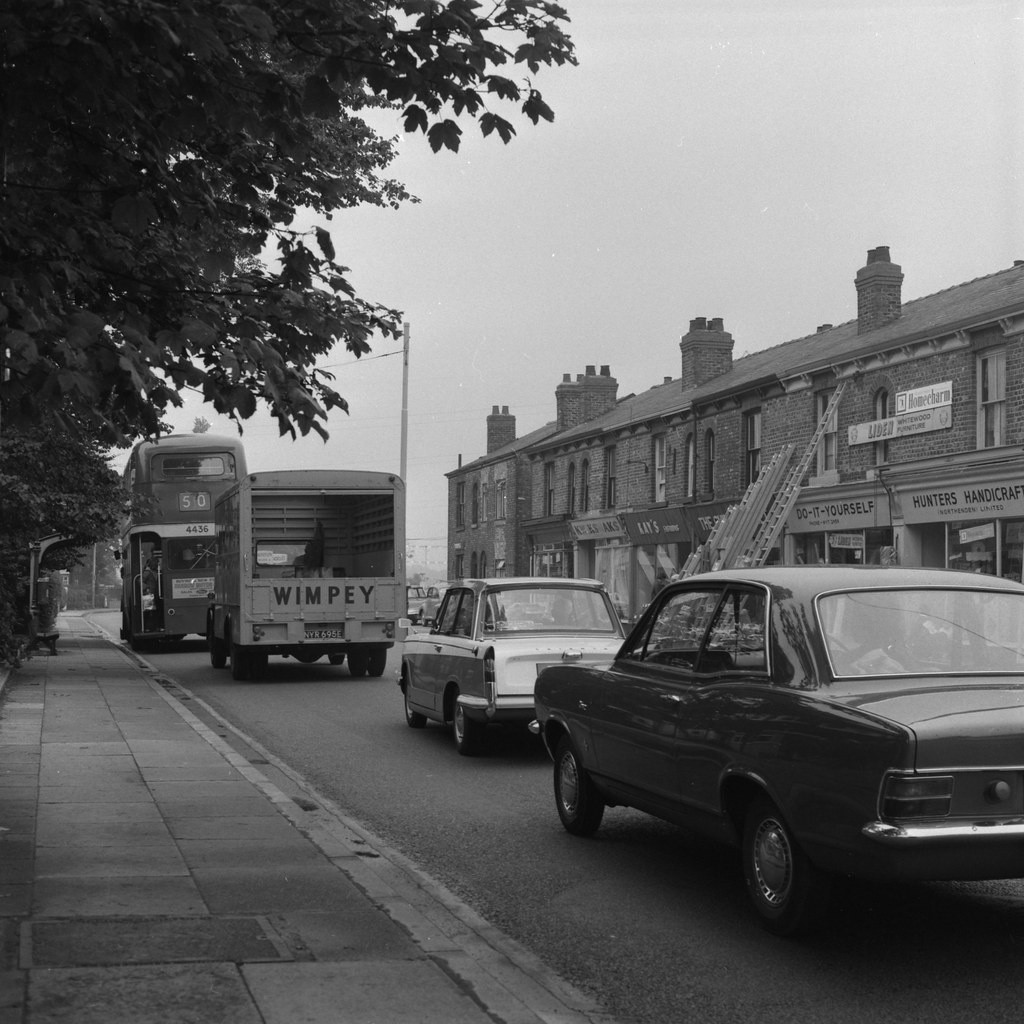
[113,433,249,652]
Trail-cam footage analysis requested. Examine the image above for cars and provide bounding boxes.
[407,582,455,627]
[397,573,643,756]
[528,565,1024,942]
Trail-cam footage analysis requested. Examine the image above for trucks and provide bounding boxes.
[193,468,411,680]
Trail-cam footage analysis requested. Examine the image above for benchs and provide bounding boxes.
[30,620,60,656]
